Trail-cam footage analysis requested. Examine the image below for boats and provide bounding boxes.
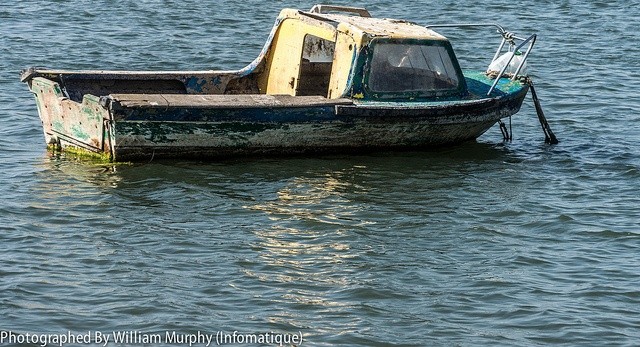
[21,2,537,183]
